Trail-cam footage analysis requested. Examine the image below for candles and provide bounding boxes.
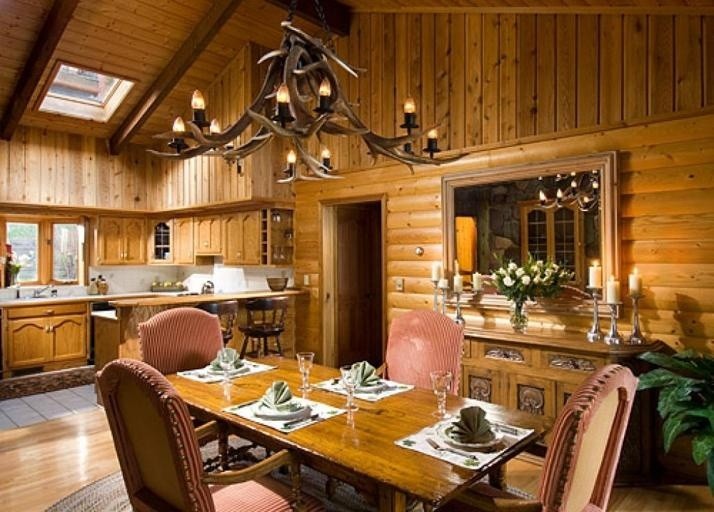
[589,267,601,287]
[472,271,481,288]
[628,273,641,295]
[431,262,441,281]
[606,280,620,302]
[439,279,448,287]
[453,274,463,294]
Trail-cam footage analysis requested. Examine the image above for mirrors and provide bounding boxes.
[439,149,624,320]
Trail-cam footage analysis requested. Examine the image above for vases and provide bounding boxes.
[510,297,529,335]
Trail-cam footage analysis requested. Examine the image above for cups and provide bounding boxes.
[50,286,56,298]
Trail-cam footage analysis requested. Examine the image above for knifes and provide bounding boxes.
[277,414,321,430]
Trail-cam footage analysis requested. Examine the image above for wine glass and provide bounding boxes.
[340,366,361,414]
[429,369,454,420]
[218,348,237,389]
[294,352,315,394]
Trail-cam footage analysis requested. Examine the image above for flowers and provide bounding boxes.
[490,250,575,312]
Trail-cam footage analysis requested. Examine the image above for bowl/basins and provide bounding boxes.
[265,277,288,292]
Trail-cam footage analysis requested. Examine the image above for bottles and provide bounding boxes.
[16,286,20,298]
[87,277,98,296]
[97,274,110,296]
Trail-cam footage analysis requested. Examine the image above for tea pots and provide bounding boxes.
[201,281,214,294]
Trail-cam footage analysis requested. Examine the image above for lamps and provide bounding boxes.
[536,168,601,212]
[143,0,469,184]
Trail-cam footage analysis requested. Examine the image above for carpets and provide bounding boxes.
[38,436,537,510]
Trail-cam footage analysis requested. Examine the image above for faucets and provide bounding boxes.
[34,284,55,297]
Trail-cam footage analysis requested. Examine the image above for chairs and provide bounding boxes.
[135,307,224,376]
[432,363,638,512]
[97,358,328,512]
[193,300,237,348]
[237,296,289,358]
[373,309,463,395]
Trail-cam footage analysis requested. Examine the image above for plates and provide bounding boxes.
[202,363,249,375]
[440,423,505,450]
[256,404,311,418]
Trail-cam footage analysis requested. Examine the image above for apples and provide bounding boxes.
[152,281,184,291]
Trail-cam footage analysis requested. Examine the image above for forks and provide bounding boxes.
[424,435,477,462]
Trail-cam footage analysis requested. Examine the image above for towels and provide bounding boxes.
[209,347,244,371]
[260,380,301,411]
[338,360,380,386]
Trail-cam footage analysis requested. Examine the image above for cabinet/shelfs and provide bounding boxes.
[88,216,145,265]
[457,320,666,464]
[172,216,213,266]
[193,214,222,256]
[0,301,91,370]
[222,208,295,269]
[147,218,171,266]
[516,199,588,291]
